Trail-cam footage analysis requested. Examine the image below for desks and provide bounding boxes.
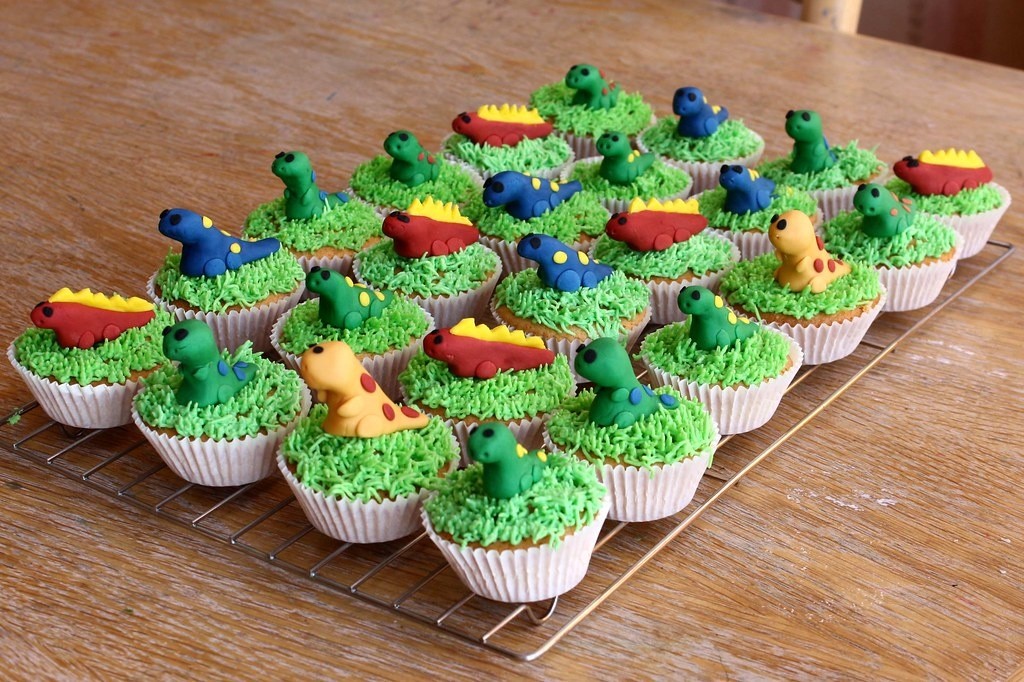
[3,3,1024,681]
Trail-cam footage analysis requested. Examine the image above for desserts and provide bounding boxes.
[7,64,1013,603]
